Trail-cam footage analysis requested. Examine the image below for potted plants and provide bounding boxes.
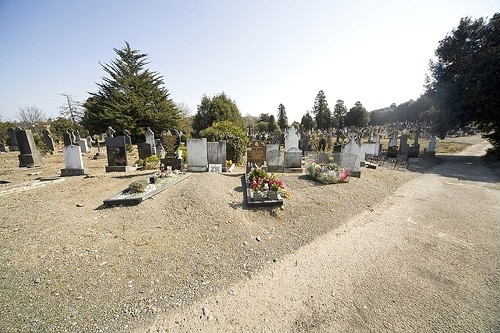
[129,154,176,192]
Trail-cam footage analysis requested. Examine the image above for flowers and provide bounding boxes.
[248,166,285,193]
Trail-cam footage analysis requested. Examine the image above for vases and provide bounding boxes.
[252,189,279,201]
